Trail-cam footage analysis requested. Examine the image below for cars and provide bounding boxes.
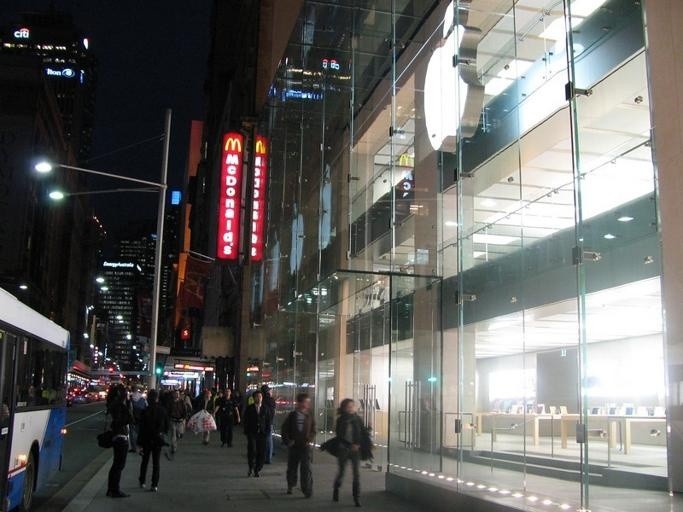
[65,381,147,408]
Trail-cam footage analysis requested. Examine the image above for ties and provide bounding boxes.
[257,406,260,414]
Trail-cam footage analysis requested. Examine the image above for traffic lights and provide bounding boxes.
[155,362,163,376]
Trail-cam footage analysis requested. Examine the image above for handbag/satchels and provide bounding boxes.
[97,430,115,449]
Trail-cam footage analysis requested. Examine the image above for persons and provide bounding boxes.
[241,390,272,478]
[319,398,375,508]
[22,384,42,407]
[259,383,276,464]
[102,383,131,497]
[279,392,317,500]
[133,387,170,495]
[122,381,241,462]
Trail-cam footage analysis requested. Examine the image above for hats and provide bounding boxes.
[261,385,272,394]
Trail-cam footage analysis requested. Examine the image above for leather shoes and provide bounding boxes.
[333,488,339,501]
[129,449,136,453]
[150,487,158,492]
[106,490,127,498]
[139,452,144,456]
[354,496,360,506]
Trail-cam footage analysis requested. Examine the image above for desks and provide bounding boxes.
[476,412,667,455]
[357,408,388,441]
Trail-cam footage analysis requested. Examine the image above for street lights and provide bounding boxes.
[34,159,168,396]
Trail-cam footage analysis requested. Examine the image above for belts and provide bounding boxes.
[116,434,129,440]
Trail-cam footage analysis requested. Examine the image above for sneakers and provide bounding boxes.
[248,459,272,478]
[287,486,293,495]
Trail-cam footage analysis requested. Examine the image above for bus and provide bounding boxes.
[0,286,73,511]
